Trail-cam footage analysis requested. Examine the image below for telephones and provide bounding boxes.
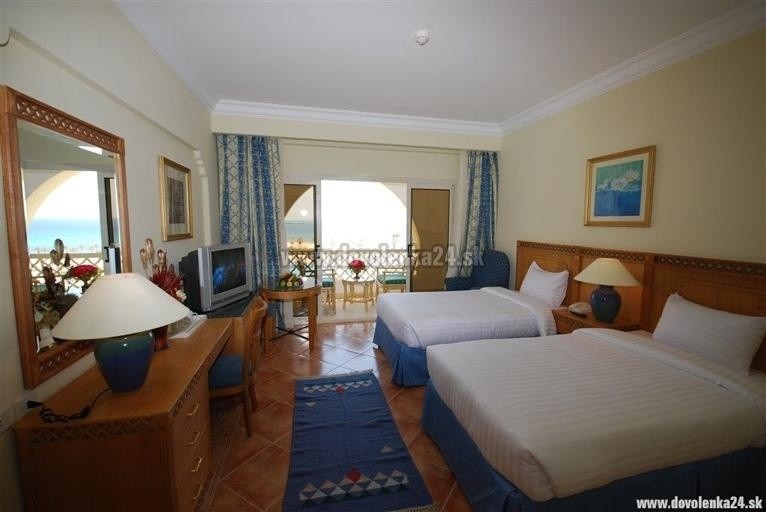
[569,302,591,315]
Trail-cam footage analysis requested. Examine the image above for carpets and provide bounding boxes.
[282,369,439,512]
[193,397,246,511]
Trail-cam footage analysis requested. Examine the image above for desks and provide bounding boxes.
[11,317,235,512]
[260,274,322,353]
[342,278,375,311]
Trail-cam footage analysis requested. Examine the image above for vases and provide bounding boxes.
[353,269,361,279]
[150,323,169,352]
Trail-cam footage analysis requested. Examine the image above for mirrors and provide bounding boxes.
[0,83,132,392]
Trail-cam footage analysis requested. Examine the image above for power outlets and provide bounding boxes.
[0,404,16,435]
[13,390,39,420]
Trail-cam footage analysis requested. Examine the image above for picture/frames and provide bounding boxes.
[583,144,656,227]
[158,155,195,243]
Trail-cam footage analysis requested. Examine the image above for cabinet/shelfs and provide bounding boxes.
[211,288,261,356]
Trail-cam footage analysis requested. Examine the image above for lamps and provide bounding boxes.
[51,273,191,395]
[573,258,640,323]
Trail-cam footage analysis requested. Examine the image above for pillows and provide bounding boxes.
[520,261,570,308]
[651,290,766,376]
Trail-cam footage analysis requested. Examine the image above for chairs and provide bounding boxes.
[323,268,336,314]
[444,251,510,289]
[210,297,268,436]
[376,266,407,302]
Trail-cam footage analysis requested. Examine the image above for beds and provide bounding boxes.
[422,254,766,512]
[372,240,581,386]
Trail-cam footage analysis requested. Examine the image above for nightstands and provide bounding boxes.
[551,308,640,333]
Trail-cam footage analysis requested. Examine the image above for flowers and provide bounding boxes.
[348,259,365,274]
[43,238,71,297]
[139,238,187,304]
[65,264,99,295]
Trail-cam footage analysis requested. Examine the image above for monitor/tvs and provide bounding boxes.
[108,247,121,274]
[179,242,252,314]
